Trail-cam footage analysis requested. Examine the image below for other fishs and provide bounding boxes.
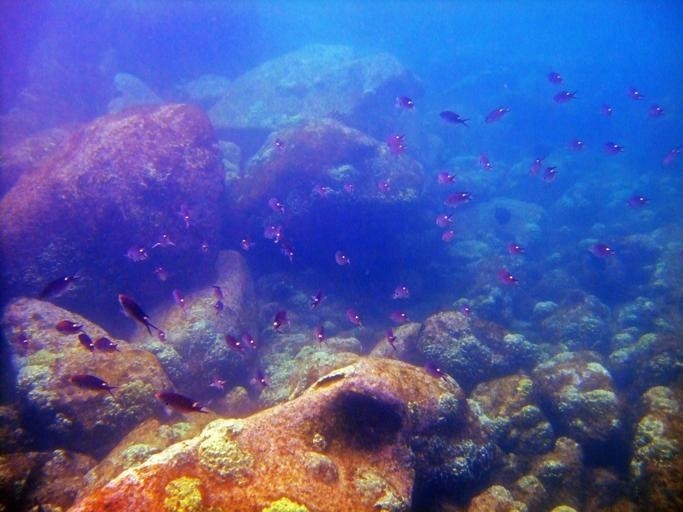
[37,69,682,414]
